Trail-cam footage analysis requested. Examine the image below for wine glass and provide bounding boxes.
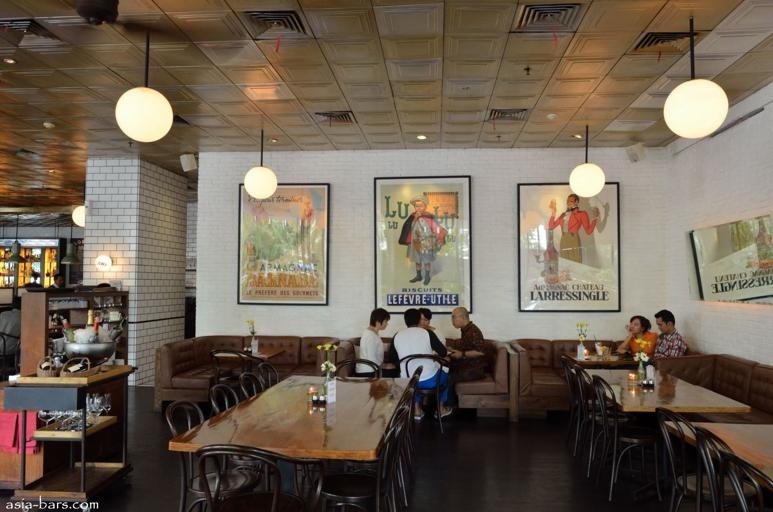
[37,393,111,430]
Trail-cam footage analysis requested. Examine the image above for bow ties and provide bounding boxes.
[567,207,574,211]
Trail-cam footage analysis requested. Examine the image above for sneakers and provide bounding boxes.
[414,409,424,420]
[434,406,452,419]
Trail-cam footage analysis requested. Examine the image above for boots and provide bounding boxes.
[424,271,430,285]
[410,270,422,282]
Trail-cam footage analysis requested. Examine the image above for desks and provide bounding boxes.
[4,362,138,504]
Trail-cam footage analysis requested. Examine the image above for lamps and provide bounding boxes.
[243,130,279,203]
[569,125,606,198]
[3,214,27,263]
[662,17,729,142]
[114,30,174,146]
[71,181,86,228]
[61,219,81,265]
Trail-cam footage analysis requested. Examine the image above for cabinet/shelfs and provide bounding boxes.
[20,289,129,466]
[0,379,72,491]
[0,247,58,287]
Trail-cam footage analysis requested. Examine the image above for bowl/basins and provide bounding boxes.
[52,340,114,368]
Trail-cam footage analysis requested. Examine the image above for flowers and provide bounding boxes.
[576,320,590,347]
[317,344,339,380]
[247,320,259,342]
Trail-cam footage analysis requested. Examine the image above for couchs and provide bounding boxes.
[506,338,707,422]
[336,335,520,424]
[152,330,339,412]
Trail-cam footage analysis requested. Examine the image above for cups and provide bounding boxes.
[306,392,318,407]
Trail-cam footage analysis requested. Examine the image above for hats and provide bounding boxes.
[410,195,429,205]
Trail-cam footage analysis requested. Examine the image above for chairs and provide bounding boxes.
[0,331,20,383]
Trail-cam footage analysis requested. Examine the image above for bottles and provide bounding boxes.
[311,395,317,410]
[61,309,101,343]
[627,363,656,392]
[582,340,620,362]
[66,358,108,373]
[318,396,325,412]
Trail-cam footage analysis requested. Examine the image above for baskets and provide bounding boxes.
[36,357,57,377]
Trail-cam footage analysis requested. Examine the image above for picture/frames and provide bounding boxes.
[691,214,773,302]
[373,175,474,317]
[516,182,623,314]
[235,182,331,308]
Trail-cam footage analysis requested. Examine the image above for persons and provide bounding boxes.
[616,316,658,358]
[0,297,20,354]
[549,194,600,263]
[398,195,448,285]
[419,309,446,354]
[48,274,64,288]
[388,309,452,420]
[353,308,395,377]
[654,310,688,359]
[444,307,488,407]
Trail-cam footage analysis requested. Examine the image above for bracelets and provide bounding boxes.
[463,350,466,359]
